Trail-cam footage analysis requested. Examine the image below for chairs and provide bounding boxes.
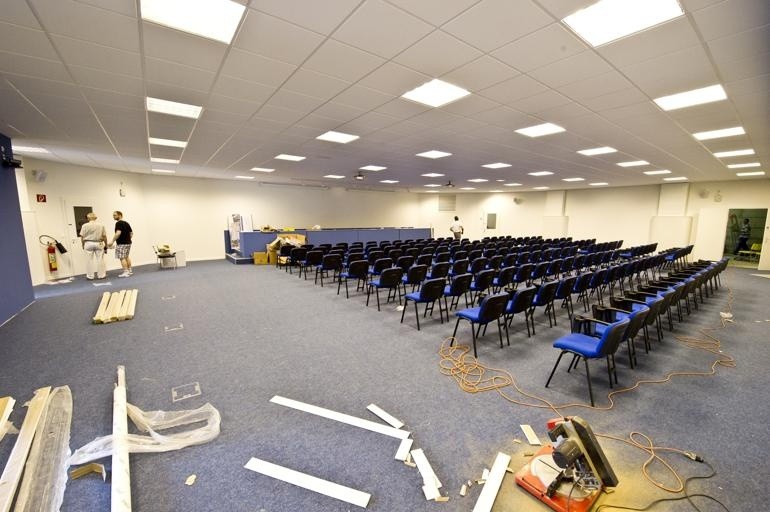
[401,240,731,410]
[152,242,178,271]
[276,233,658,312]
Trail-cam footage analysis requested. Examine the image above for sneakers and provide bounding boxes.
[119,272,132,277]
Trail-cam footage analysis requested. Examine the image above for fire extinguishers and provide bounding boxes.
[39,234,67,272]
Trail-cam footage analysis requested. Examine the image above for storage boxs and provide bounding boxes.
[251,251,269,264]
[268,251,277,265]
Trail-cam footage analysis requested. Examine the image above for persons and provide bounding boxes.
[108,210,134,278]
[79,212,107,280]
[733,218,752,255]
[449,215,464,239]
